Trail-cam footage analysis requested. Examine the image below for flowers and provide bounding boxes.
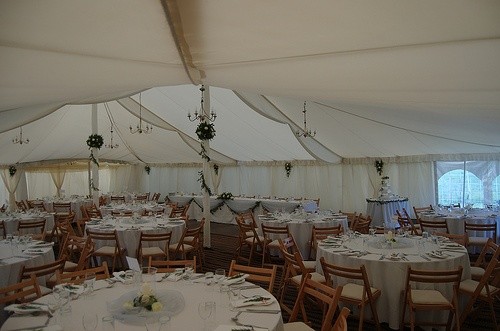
[125,283,163,315]
[384,231,396,246]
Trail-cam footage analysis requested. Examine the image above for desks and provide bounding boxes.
[167,195,317,227]
[365,197,409,229]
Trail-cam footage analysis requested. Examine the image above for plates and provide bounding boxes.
[23,248,52,254]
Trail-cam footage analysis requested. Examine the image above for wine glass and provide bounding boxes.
[215,269,225,294]
[198,304,211,331]
[47,299,60,326]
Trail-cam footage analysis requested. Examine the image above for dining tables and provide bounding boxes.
[317,233,471,297]
[2,272,284,331]
[259,214,349,262]
[0,210,56,237]
[83,216,186,255]
[0,238,55,288]
[429,207,500,239]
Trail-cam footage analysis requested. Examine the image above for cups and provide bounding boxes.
[83,280,93,297]
[125,270,141,292]
[417,240,425,256]
[86,272,97,281]
[102,317,114,331]
[141,267,157,292]
[60,292,72,314]
[82,313,98,331]
[205,302,216,322]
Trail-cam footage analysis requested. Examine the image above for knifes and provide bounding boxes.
[246,309,280,311]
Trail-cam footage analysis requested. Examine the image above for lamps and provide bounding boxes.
[187,85,218,124]
[12,127,30,144]
[103,126,120,150]
[127,92,153,134]
[296,100,316,139]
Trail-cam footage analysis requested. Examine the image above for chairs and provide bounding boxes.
[0,192,499,331]
